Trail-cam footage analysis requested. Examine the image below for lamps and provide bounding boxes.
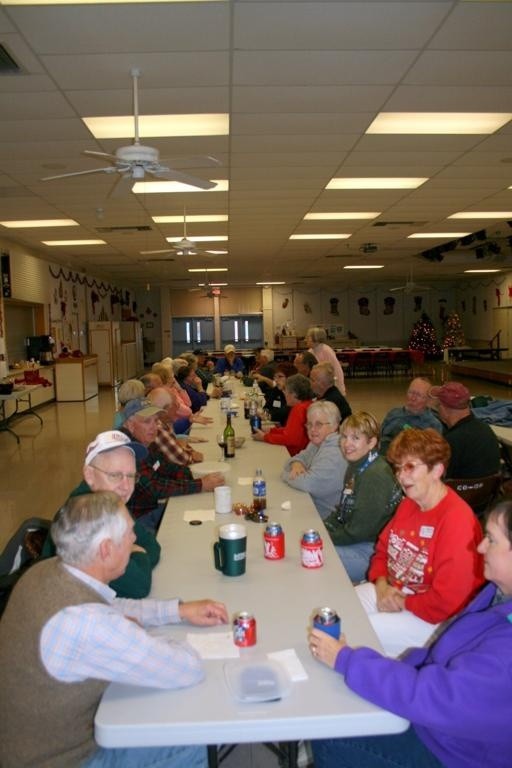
[133,165,145,180]
[183,249,188,256]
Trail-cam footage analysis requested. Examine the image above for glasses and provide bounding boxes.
[304,422,329,430]
[392,462,423,476]
[89,464,141,484]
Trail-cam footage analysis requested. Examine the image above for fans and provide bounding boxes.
[139,203,231,260]
[41,68,222,200]
[388,263,435,294]
[187,271,232,293]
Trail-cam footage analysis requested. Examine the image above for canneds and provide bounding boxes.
[234,614,254,628]
[303,529,321,543]
[266,523,283,535]
[317,606,338,625]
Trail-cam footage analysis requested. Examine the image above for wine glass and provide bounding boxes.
[217,434,224,462]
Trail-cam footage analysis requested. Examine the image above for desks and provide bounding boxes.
[469,393,512,470]
[179,347,425,371]
[0,384,43,444]
[92,374,411,768]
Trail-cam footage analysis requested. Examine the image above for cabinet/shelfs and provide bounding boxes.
[87,320,145,388]
[54,354,99,403]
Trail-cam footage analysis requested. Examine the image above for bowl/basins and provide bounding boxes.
[1,383,14,395]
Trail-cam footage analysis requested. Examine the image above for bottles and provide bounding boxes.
[252,469,266,509]
[224,414,235,457]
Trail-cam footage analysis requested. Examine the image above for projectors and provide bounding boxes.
[360,246,378,254]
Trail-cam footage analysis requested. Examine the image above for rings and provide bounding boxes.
[312,646,316,653]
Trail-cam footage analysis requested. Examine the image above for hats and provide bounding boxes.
[124,397,168,420]
[428,381,469,408]
[224,344,235,354]
[193,348,208,356]
[85,430,149,466]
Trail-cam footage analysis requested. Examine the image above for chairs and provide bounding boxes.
[213,346,414,379]
[441,468,504,520]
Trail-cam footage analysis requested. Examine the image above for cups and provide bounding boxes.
[218,369,269,433]
[234,437,245,448]
[214,486,232,514]
[213,525,247,575]
[12,358,41,368]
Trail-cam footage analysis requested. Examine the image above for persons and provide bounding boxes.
[113,346,222,529]
[283,399,404,580]
[42,430,160,599]
[215,344,243,373]
[0,491,228,768]
[238,328,353,457]
[308,502,512,768]
[322,376,502,657]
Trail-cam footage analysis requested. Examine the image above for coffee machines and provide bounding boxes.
[25,336,55,365]
